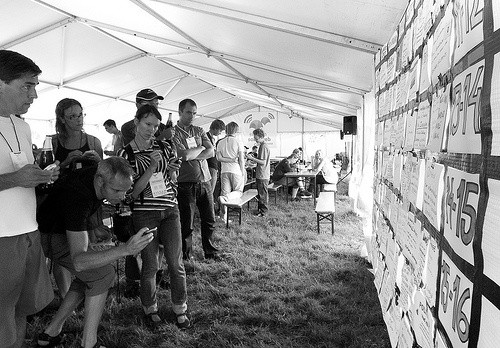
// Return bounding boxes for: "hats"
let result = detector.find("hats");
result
[136,88,164,103]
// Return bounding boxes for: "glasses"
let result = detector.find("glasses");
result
[61,113,86,120]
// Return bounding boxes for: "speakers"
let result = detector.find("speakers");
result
[344,115,357,135]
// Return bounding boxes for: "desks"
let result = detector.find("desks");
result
[284,164,321,205]
[246,161,279,181]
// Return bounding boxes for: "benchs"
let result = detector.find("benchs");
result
[221,171,337,236]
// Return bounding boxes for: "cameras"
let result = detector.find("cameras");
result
[144,227,158,239]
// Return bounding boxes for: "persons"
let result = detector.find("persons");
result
[0,50,60,348]
[36,88,187,348]
[206,119,258,223]
[174,99,226,263]
[246,128,270,218]
[272,147,338,202]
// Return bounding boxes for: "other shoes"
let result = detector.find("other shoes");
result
[147,313,162,324]
[205,252,224,261]
[79,342,107,348]
[291,198,302,202]
[38,332,65,345]
[126,283,140,297]
[176,312,191,328]
[300,191,312,196]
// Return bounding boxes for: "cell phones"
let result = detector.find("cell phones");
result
[43,163,57,171]
[81,150,94,158]
[171,157,183,164]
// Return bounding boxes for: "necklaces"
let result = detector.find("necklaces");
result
[62,131,83,154]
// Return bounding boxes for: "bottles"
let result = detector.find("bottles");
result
[166,113,174,142]
[297,162,299,172]
[40,135,54,189]
